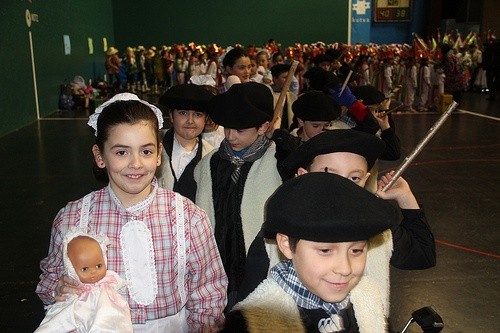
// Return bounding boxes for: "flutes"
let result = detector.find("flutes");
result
[375,101,459,192]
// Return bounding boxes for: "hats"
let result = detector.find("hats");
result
[292,129,384,170]
[207,82,274,129]
[159,83,215,117]
[291,91,341,122]
[350,85,385,105]
[262,171,401,243]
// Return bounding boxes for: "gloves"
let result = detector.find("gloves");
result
[333,86,355,106]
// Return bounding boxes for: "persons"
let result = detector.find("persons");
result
[217,171,400,333]
[33,225,135,333]
[35,92,229,333]
[288,89,352,142]
[59,27,500,115]
[269,64,295,129]
[228,129,436,308]
[199,86,226,149]
[158,83,216,191]
[327,82,358,129]
[172,82,306,288]
[350,84,400,160]
